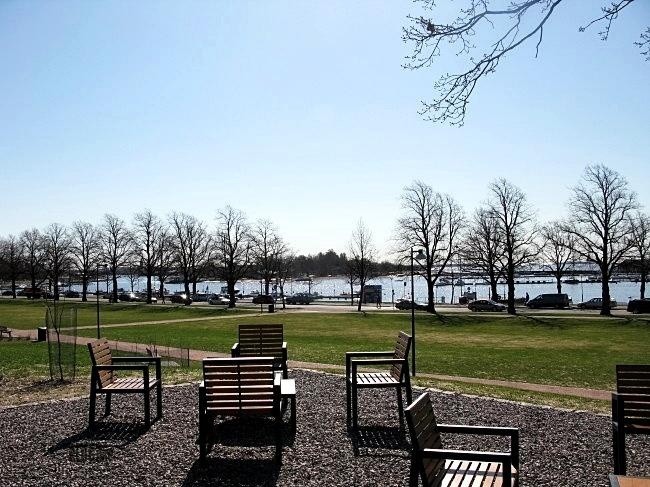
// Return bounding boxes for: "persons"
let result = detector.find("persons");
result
[526,292,528,302]
[343,291,347,302]
[162,295,166,305]
[376,294,381,308]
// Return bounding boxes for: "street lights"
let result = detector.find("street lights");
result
[411,247,425,377]
[96,263,109,339]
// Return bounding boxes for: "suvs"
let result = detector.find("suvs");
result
[284,291,314,305]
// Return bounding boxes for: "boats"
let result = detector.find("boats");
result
[338,290,360,297]
[394,268,466,287]
[532,269,650,285]
[16,275,284,300]
[294,274,314,281]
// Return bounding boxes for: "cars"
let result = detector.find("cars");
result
[1,290,13,297]
[251,295,276,305]
[576,297,617,310]
[135,295,158,304]
[395,299,429,311]
[169,293,194,305]
[208,296,230,305]
[466,298,506,312]
[626,296,650,314]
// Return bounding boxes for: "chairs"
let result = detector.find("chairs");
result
[228,321,291,378]
[82,334,162,432]
[606,359,650,472]
[401,389,526,487]
[194,356,287,461]
[343,328,417,445]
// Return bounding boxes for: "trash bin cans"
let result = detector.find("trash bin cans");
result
[269,305,274,313]
[38,327,47,341]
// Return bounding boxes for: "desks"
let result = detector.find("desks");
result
[607,471,647,487]
[271,368,297,422]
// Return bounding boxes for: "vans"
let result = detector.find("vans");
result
[526,292,571,309]
